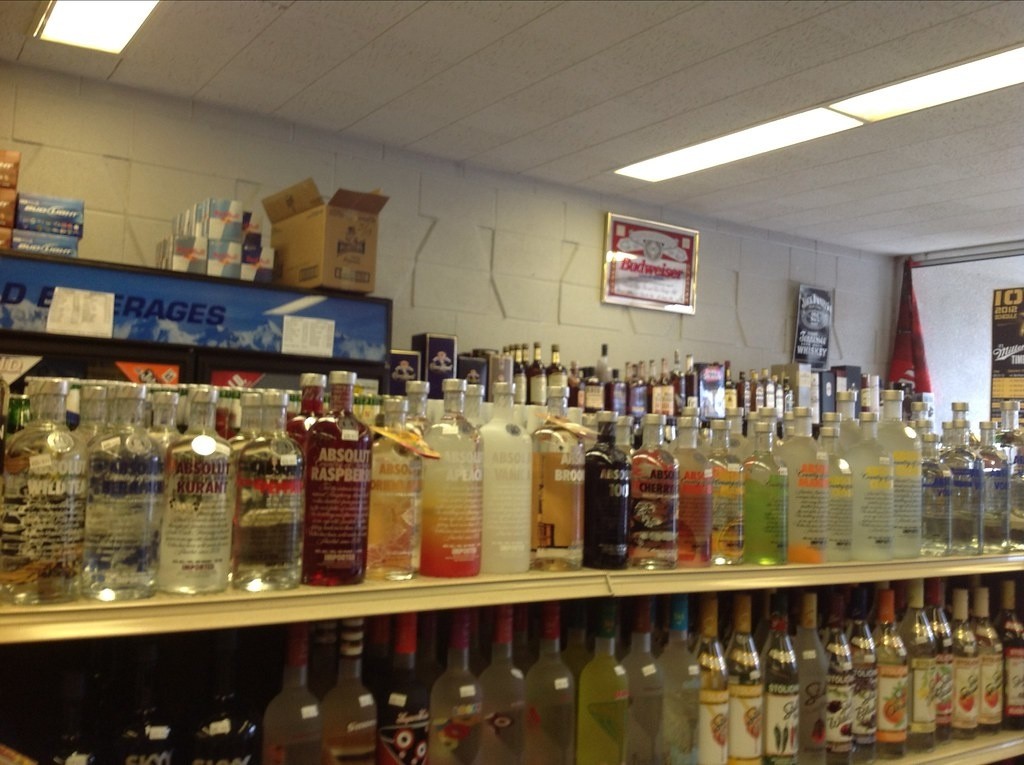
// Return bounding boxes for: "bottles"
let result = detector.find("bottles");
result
[0,563,1024,765]
[0,341,1024,602]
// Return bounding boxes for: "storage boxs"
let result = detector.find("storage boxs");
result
[0,227,12,252]
[457,356,488,402]
[390,348,421,396]
[15,190,85,238]
[11,229,79,258]
[0,188,18,228]
[412,333,458,400]
[0,149,22,189]
[161,196,257,283]
[261,177,391,293]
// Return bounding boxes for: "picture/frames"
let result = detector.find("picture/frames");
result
[598,212,700,316]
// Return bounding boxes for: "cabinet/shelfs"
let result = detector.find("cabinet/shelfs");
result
[0,546,1024,765]
